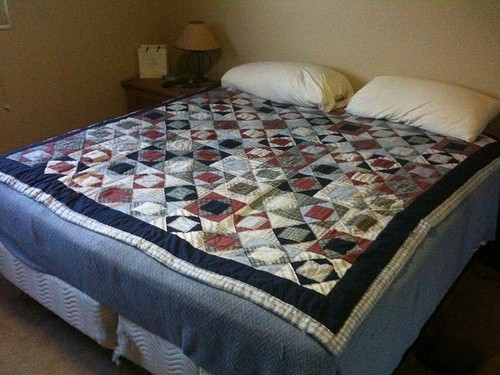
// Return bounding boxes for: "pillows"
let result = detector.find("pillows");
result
[220,60,356,114]
[344,73,498,145]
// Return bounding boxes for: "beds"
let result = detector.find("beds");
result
[0,88,500,375]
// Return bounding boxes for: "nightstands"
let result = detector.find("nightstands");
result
[119,75,221,113]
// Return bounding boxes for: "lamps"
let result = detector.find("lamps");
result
[174,20,220,89]
